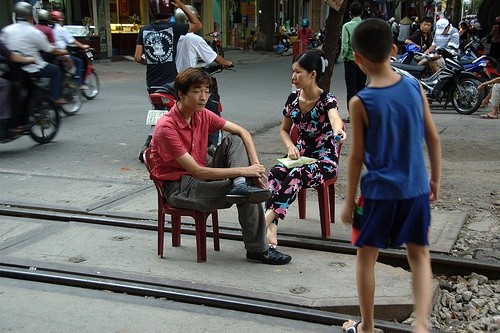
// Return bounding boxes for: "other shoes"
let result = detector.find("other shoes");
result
[49,97,70,106]
[343,116,350,123]
[0,122,25,134]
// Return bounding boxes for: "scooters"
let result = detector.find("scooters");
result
[276,25,326,52]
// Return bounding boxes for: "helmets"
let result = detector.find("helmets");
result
[50,11,65,21]
[13,1,33,20]
[38,10,50,21]
[302,19,309,26]
[175,5,200,24]
[148,0,175,18]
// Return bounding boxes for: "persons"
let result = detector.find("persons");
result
[133,0,232,163]
[264,48,346,249]
[341,19,441,333]
[298,19,315,50]
[386,16,500,119]
[149,67,292,265]
[0,1,90,132]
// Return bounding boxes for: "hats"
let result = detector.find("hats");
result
[436,18,449,34]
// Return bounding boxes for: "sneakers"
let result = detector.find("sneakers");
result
[246,245,292,265]
[226,183,271,204]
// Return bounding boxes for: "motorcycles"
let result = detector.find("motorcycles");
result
[389,37,498,115]
[0,43,99,144]
[146,63,237,148]
[203,31,225,58]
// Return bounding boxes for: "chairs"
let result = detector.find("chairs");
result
[291,119,346,238]
[142,147,221,262]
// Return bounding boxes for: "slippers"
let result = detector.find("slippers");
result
[343,320,361,333]
[480,113,499,119]
